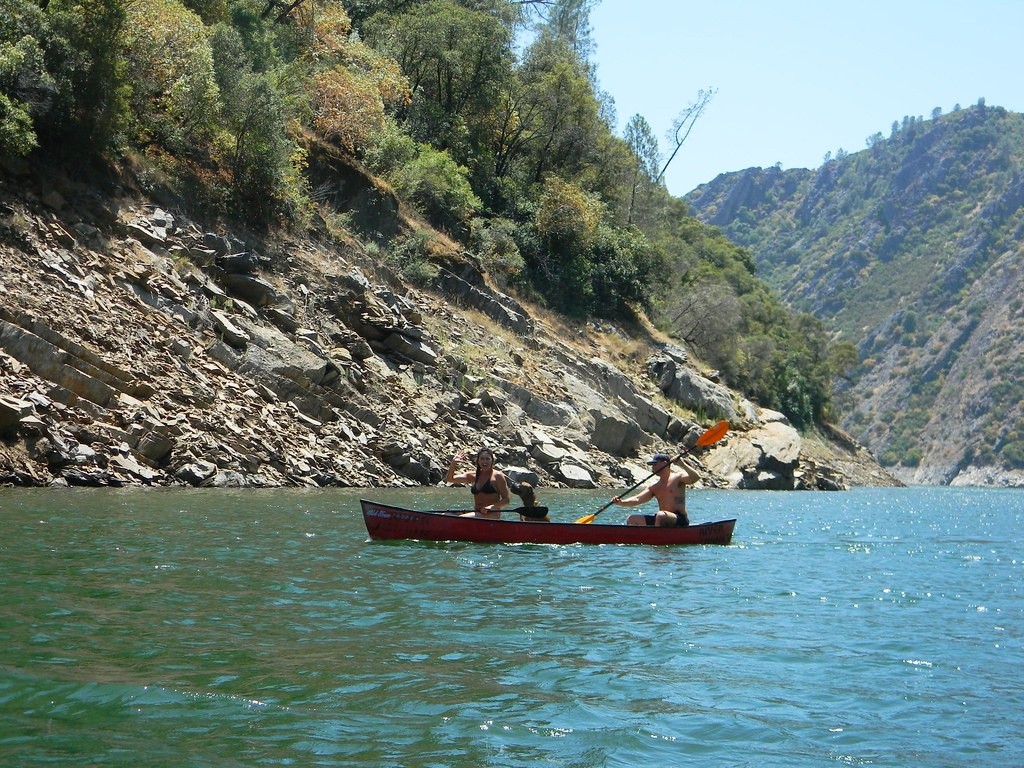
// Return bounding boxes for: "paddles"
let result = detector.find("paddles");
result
[428,506,549,518]
[574,420,730,524]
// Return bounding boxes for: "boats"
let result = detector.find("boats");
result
[359,499,737,545]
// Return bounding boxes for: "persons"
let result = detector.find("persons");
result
[612,454,701,527]
[443,447,510,518]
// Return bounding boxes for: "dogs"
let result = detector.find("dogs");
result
[510,481,551,522]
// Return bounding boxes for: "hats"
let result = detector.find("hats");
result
[647,454,669,465]
